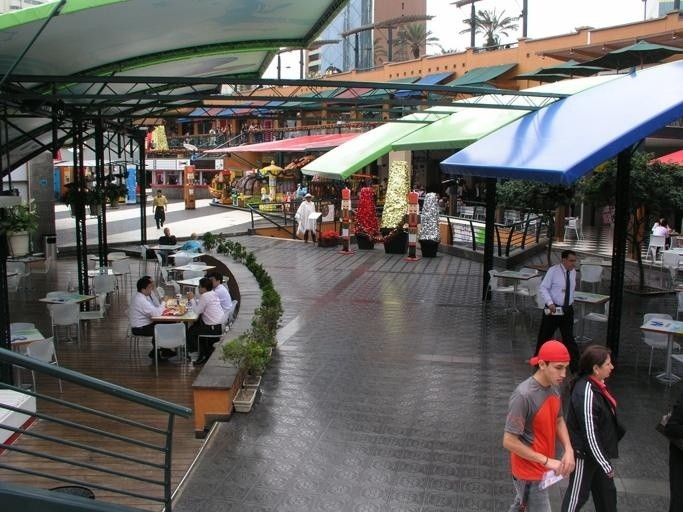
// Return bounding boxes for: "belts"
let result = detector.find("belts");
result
[205,324,221,330]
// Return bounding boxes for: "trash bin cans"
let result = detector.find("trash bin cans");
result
[44,234,57,260]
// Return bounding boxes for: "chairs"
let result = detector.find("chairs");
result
[488,267,543,308]
[635,229,682,386]
[458,198,583,242]
[582,300,610,344]
[554,314,579,341]
[579,265,605,293]
[6,246,238,394]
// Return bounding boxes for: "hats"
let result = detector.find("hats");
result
[529,340,572,367]
[304,193,314,198]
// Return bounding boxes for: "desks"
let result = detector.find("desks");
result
[573,291,610,346]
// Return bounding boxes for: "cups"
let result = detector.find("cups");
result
[176,294,182,304]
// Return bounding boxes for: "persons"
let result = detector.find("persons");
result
[446,179,484,202]
[294,193,318,244]
[159,226,178,245]
[153,190,169,228]
[561,344,620,512]
[184,233,201,250]
[208,124,231,146]
[502,339,576,512]
[651,217,673,253]
[128,271,232,365]
[239,121,265,144]
[534,250,583,374]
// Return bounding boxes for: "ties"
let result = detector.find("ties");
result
[563,270,570,316]
[149,295,153,302]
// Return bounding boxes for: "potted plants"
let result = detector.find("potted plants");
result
[204,231,283,413]
[459,9,523,51]
[4,204,40,258]
[392,24,445,58]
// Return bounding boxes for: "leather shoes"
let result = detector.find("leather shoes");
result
[149,349,168,361]
[169,350,177,358]
[195,352,209,365]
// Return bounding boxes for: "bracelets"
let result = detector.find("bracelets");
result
[542,455,549,467]
